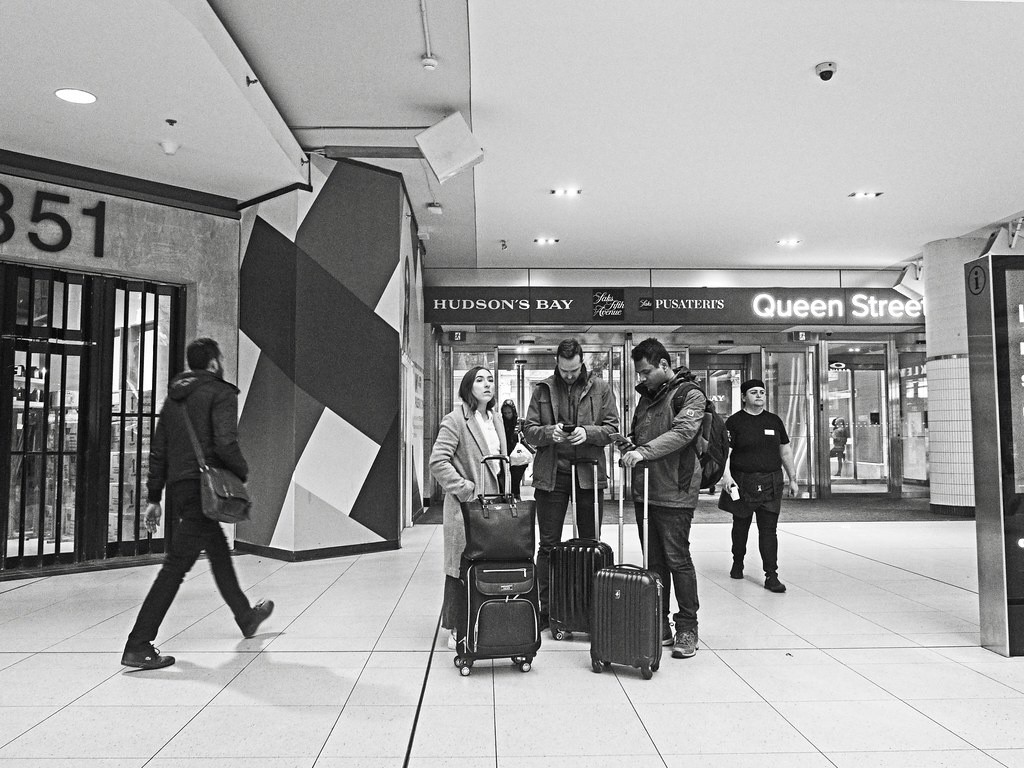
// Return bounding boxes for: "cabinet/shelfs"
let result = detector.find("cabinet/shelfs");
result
[25,406,151,543]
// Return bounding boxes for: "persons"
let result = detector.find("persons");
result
[120,338,274,670]
[829,417,847,477]
[501,399,537,502]
[721,379,798,593]
[523,339,619,631]
[614,339,707,659]
[428,367,513,650]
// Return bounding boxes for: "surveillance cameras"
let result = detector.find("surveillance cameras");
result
[826,331,832,336]
[815,62,837,82]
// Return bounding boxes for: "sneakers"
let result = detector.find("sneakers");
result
[672,625,700,658]
[121,643,174,670]
[662,628,673,645]
[730,560,744,579]
[242,599,274,639]
[764,576,786,593]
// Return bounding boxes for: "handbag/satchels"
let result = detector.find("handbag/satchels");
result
[509,433,534,466]
[461,493,536,561]
[200,467,251,525]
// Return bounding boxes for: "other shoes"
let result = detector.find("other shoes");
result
[710,487,716,494]
[842,453,846,460]
[834,473,841,476]
[448,628,457,652]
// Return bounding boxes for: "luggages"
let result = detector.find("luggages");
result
[549,458,615,642]
[454,455,542,678]
[589,459,664,680]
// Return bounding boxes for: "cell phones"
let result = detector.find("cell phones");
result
[562,425,575,434]
[608,433,633,445]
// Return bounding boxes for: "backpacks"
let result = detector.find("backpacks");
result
[674,382,729,489]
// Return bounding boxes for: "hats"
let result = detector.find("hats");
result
[740,379,763,392]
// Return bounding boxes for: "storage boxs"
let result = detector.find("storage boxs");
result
[44,390,153,538]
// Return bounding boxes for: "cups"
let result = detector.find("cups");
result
[730,487,740,501]
